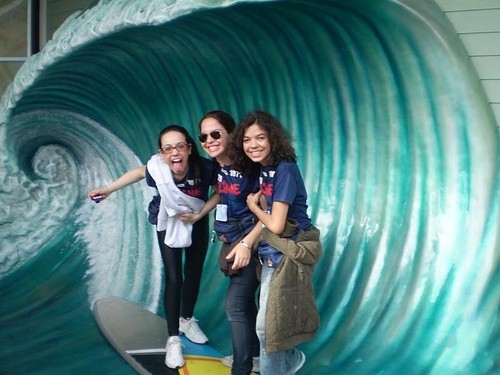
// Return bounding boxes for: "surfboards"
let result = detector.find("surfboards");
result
[93,296,258,375]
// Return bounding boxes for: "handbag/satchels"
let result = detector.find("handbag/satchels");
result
[213,214,257,244]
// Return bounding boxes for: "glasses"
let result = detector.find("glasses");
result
[162,142,190,154]
[198,129,225,145]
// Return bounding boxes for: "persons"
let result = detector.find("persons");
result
[226,109,322,375]
[199,110,262,375]
[86,124,221,369]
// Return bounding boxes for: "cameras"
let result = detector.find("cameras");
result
[90,194,107,203]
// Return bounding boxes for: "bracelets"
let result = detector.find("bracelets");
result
[240,241,252,250]
[104,186,111,195]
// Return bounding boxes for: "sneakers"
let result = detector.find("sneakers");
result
[165,337,185,368]
[177,315,208,343]
[220,351,261,371]
